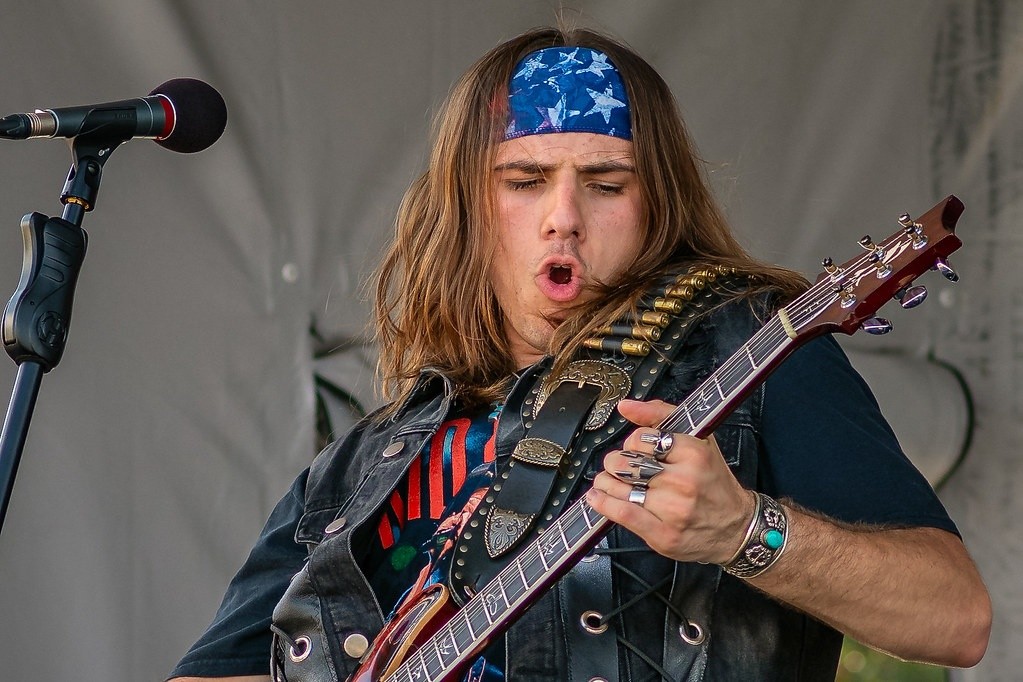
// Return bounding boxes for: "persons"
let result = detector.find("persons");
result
[163,25,993,682]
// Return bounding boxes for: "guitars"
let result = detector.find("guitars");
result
[344,194,966,682]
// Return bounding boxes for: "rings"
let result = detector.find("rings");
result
[614,428,675,507]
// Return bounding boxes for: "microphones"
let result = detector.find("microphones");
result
[0,77,228,155]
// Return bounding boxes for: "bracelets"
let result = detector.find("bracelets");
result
[719,490,789,580]
